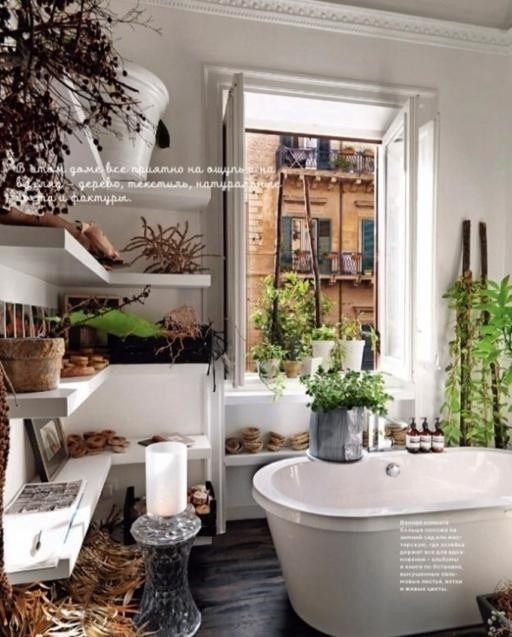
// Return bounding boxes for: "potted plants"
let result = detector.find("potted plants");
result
[250,273,395,464]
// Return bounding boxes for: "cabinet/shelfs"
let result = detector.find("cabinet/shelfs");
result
[0,48,211,586]
[224,396,420,467]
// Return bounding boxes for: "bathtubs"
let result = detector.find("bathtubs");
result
[251,448,512,637]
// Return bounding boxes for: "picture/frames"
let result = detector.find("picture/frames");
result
[24,419,70,485]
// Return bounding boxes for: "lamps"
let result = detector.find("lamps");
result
[129,443,203,637]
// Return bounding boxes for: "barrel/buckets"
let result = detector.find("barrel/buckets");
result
[309,405,365,462]
[72,62,169,182]
